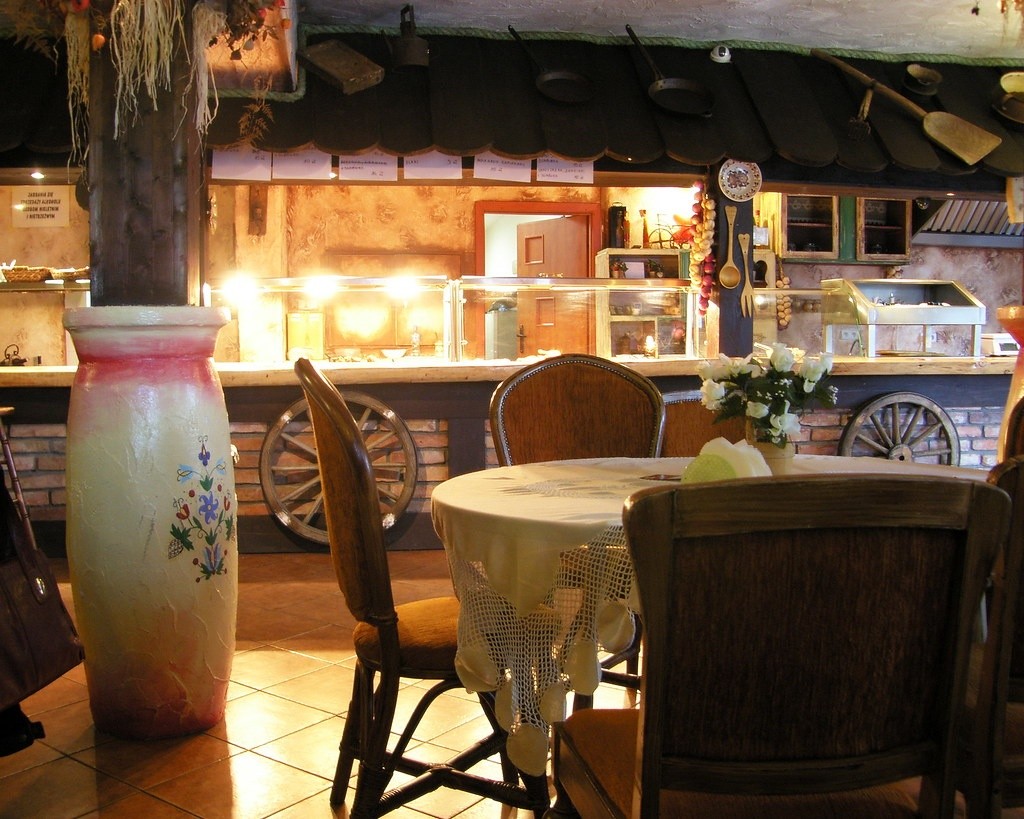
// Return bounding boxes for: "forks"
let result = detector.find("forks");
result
[736,233,758,318]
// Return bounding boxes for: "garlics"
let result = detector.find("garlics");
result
[775,276,791,327]
[792,302,820,311]
[688,198,716,291]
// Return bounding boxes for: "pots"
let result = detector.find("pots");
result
[622,22,720,120]
[504,25,597,105]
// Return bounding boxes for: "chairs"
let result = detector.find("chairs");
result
[296,358,552,818]
[550,398,1024,819]
[489,350,664,467]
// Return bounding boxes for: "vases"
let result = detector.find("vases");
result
[744,413,796,476]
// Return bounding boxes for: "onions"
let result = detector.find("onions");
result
[689,179,703,245]
[698,254,714,315]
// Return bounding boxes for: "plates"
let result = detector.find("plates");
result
[717,158,762,203]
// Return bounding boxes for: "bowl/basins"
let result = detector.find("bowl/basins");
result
[993,72,1024,123]
[380,349,408,358]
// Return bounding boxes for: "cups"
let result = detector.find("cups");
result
[904,63,942,94]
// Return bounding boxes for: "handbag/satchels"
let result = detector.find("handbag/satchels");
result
[0,479,84,710]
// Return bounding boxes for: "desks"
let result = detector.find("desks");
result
[432,457,991,768]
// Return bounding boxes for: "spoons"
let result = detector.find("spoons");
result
[719,205,741,289]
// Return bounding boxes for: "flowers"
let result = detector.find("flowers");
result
[697,341,838,448]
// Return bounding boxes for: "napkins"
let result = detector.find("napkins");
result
[700,437,774,477]
